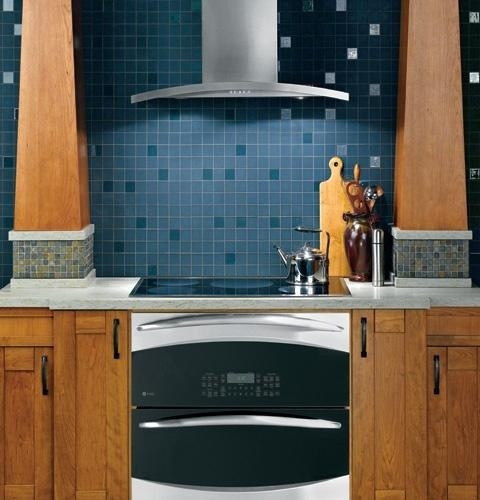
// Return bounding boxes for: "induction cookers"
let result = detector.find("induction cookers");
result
[131,273,352,298]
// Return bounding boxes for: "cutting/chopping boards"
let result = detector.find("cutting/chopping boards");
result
[318,154,354,279]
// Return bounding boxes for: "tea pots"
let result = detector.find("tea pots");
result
[274,226,331,287]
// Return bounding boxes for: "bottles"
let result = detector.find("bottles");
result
[371,228,384,286]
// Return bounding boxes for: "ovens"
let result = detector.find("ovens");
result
[130,312,353,500]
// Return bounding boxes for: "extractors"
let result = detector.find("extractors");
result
[129,1,350,107]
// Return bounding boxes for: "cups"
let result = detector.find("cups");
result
[341,211,374,283]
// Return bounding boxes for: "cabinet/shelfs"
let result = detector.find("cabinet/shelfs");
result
[350,304,427,500]
[1,306,52,500]
[425,304,480,500]
[51,310,130,500]
